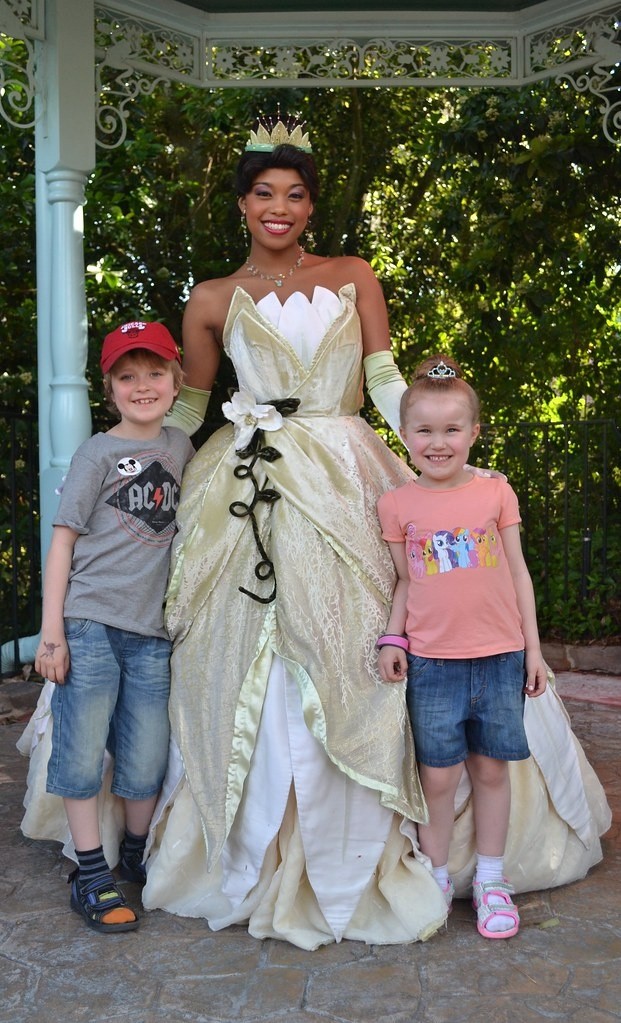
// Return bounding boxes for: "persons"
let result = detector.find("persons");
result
[35,322,197,934]
[378,356,547,938]
[16,120,611,952]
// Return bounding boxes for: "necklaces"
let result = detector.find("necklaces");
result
[246,246,304,287]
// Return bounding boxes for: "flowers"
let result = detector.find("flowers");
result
[217,389,285,450]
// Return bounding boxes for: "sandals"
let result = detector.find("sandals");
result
[430,874,455,915]
[470,872,521,939]
[118,840,151,884]
[66,866,141,932]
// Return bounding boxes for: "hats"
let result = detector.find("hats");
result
[100,321,181,374]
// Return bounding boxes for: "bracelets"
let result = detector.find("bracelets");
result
[377,634,409,651]
